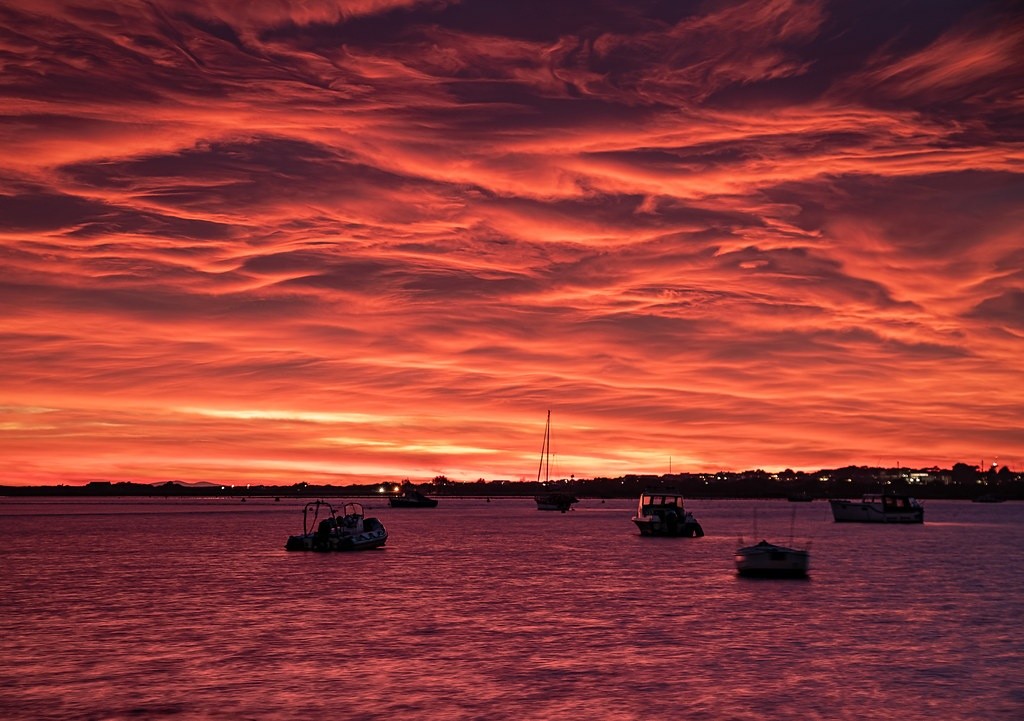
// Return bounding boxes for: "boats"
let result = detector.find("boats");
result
[788,491,812,502]
[827,494,927,524]
[632,493,704,537]
[732,540,810,576]
[285,499,388,552]
[388,491,438,507]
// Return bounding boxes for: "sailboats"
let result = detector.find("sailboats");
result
[534,410,581,510]
